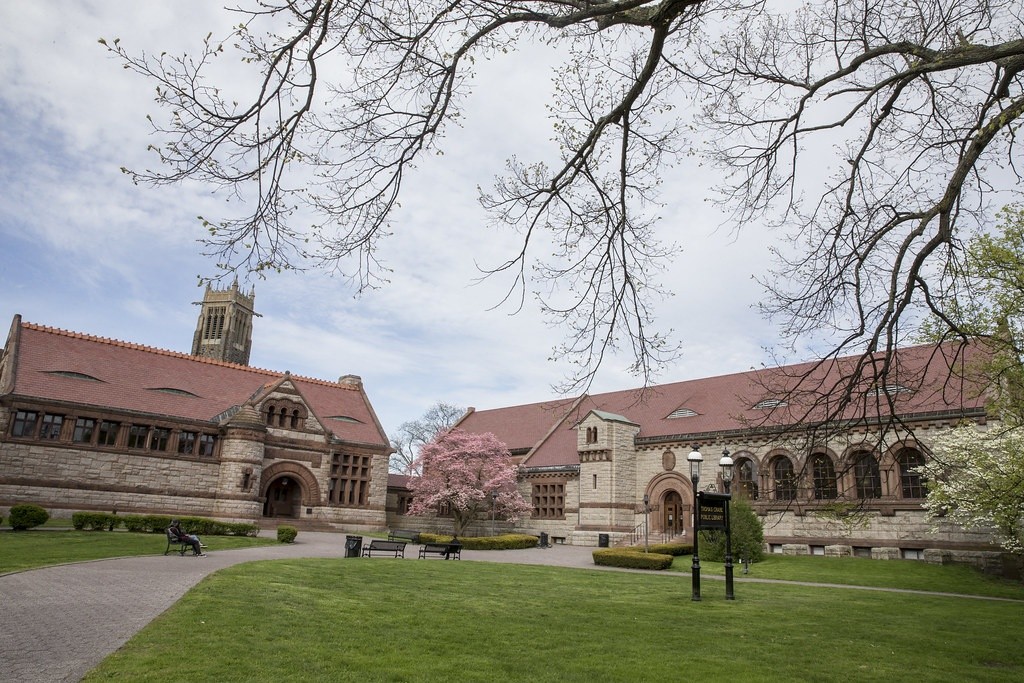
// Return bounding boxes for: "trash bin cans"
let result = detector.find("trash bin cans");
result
[345,535,363,558]
[598,532,609,548]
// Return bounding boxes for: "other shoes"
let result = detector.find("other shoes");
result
[445,558,450,560]
[200,544,207,548]
[440,553,445,555]
[196,554,206,557]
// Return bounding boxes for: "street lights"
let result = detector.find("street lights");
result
[718,445,735,600]
[687,438,704,601]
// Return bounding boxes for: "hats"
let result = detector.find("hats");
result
[173,520,178,523]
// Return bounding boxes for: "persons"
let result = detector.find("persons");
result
[168,518,207,556]
[440,533,461,560]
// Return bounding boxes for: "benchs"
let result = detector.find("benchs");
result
[164,529,193,556]
[418,543,463,561]
[552,537,565,544]
[360,539,407,558]
[387,529,421,545]
